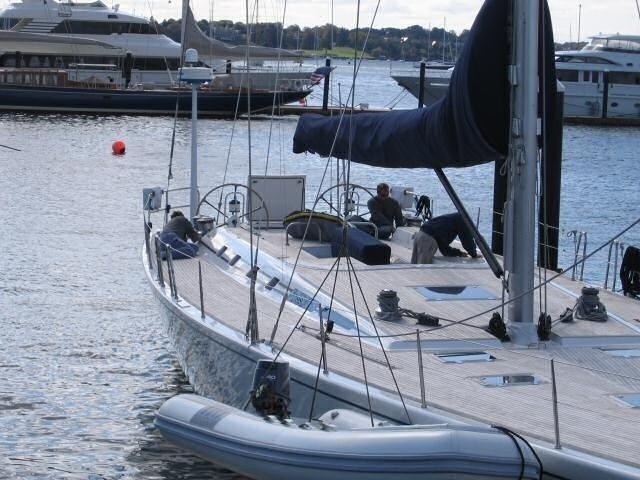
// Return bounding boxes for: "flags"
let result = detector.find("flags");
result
[310,66,334,86]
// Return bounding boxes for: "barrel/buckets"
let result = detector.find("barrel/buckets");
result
[111,141,125,154]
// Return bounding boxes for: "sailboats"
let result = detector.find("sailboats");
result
[414,15,459,70]
[136,0,640,480]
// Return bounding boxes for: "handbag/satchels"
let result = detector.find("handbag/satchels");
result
[284,212,343,241]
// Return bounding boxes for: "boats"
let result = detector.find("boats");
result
[0,0,314,92]
[0,67,316,124]
[386,30,640,125]
[147,359,545,480]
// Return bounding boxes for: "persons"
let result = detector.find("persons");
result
[410,211,483,265]
[122,51,133,89]
[156,210,202,260]
[366,182,403,241]
